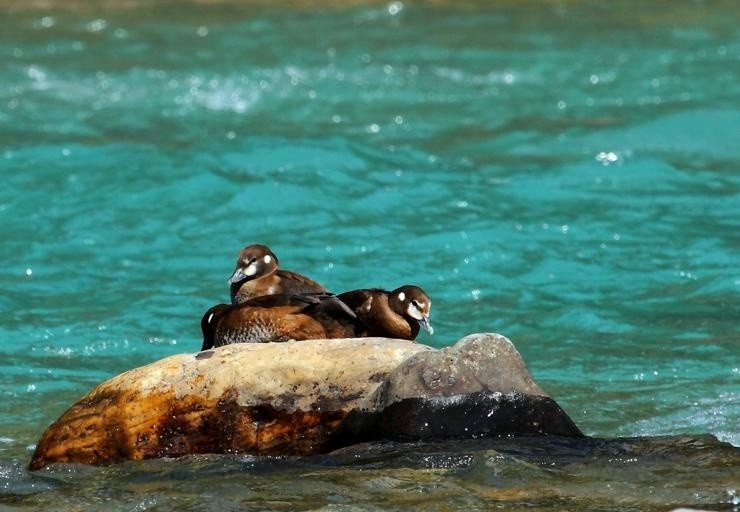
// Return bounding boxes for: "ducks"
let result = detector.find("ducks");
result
[227,244,331,304]
[200,293,357,350]
[305,283,434,340]
